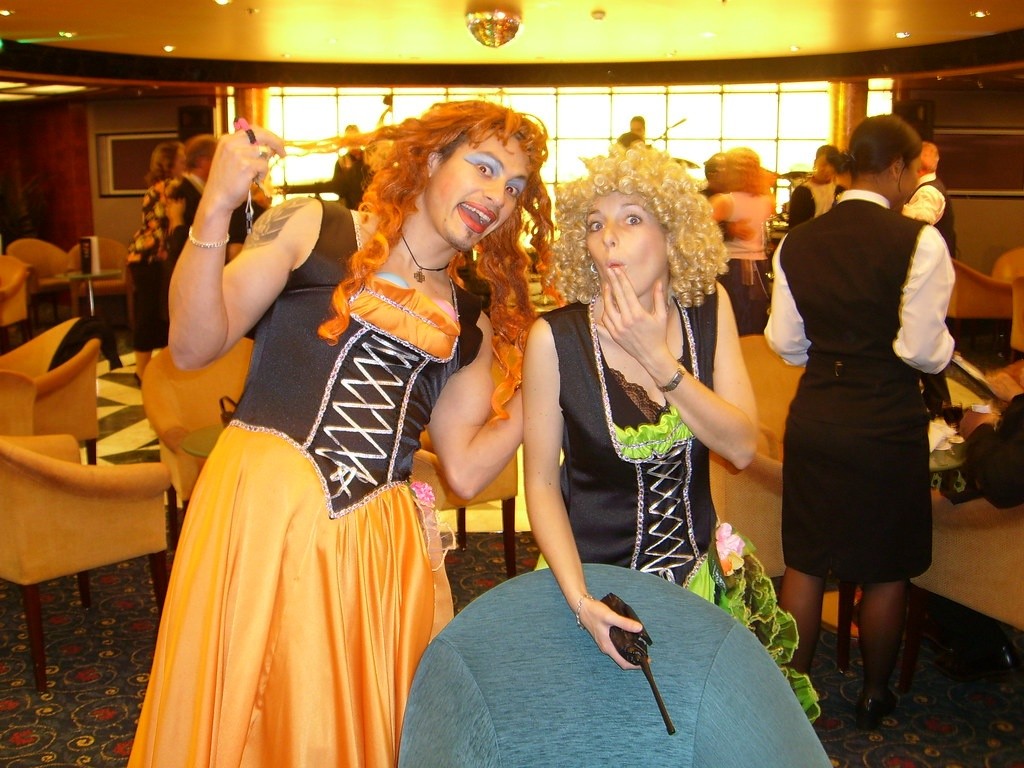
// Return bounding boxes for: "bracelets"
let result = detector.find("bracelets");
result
[575,593,595,631]
[657,365,686,393]
[188,225,230,249]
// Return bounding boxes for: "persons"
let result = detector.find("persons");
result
[521,141,761,672]
[762,114,958,732]
[120,117,956,351]
[958,358,1024,508]
[123,101,569,768]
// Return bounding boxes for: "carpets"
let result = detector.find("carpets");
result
[0,530,1024,767]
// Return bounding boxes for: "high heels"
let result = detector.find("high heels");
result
[855,689,897,727]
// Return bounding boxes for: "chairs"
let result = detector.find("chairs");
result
[410,434,517,577]
[399,563,831,768]
[0,239,253,690]
[949,246,1023,366]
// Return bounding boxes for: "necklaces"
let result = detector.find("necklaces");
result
[399,226,449,283]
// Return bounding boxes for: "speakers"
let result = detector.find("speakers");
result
[178,106,212,141]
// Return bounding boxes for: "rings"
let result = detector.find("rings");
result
[259,145,268,159]
